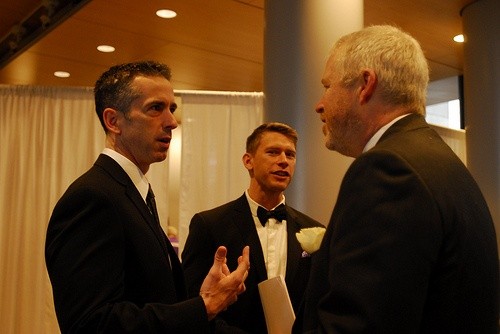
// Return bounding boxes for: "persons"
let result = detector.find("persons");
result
[292,23,500,334]
[179,121,325,334]
[45,62,250,334]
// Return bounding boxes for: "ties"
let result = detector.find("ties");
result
[146,182,160,233]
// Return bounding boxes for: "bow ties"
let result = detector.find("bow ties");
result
[257,203,288,227]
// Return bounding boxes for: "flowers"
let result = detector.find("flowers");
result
[296,226,327,258]
